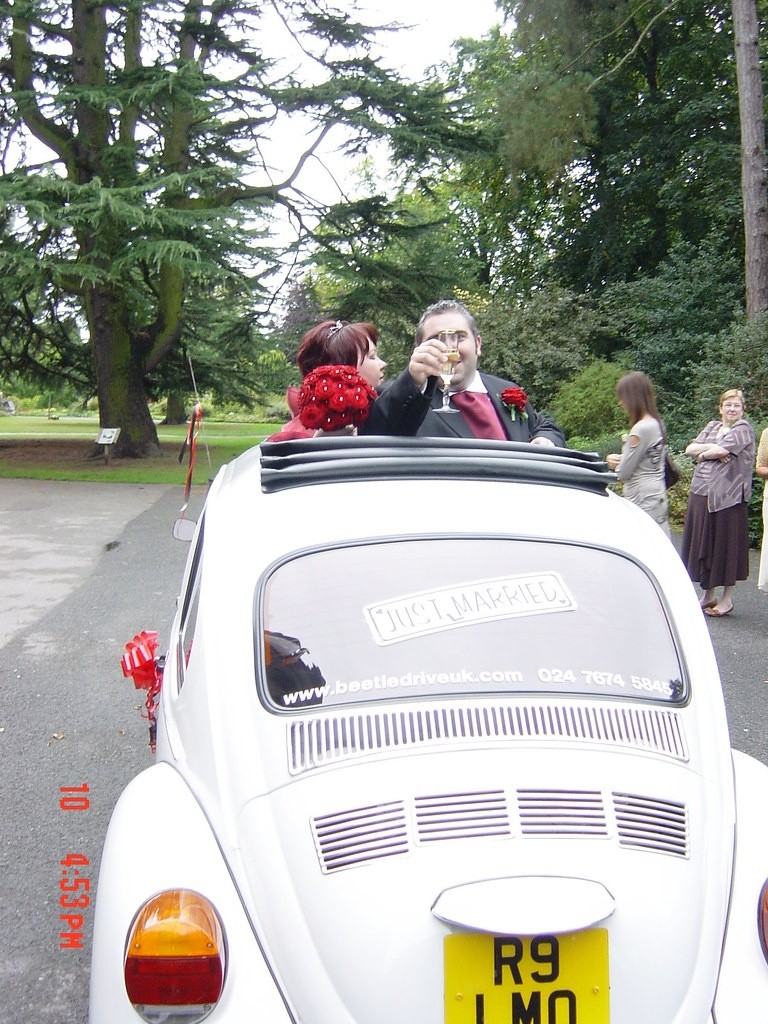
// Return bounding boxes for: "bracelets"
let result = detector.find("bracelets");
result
[701,452,705,462]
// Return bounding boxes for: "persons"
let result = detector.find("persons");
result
[357,300,566,450]
[606,371,671,543]
[755,426,768,592]
[679,389,755,617]
[260,319,388,444]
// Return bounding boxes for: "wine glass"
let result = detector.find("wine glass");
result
[431,329,461,415]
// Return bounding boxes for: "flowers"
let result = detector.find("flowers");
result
[500,386,530,423]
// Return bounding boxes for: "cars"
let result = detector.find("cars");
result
[87,424,768,1024]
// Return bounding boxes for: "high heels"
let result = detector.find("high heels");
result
[700,598,734,617]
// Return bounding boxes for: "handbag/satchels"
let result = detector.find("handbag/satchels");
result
[658,419,681,490]
